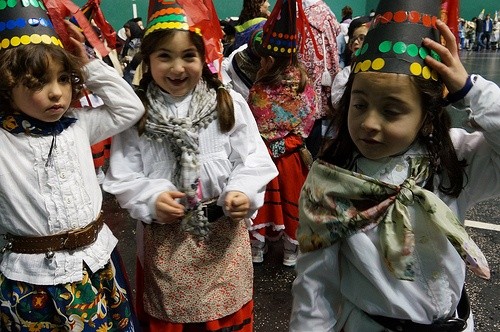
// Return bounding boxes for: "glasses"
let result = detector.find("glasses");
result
[351,35,366,44]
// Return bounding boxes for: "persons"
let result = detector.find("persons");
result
[103,1,279,331]
[0,0,145,332]
[289,0,500,332]
[68,1,500,266]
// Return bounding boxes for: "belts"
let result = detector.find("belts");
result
[363,284,470,332]
[202,200,224,223]
[0,207,105,259]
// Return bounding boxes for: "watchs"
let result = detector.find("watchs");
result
[446,74,478,103]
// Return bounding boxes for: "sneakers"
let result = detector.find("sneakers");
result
[252,247,264,263]
[283,249,298,266]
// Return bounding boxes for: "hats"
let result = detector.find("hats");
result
[351,0,444,85]
[142,0,203,41]
[68,0,101,33]
[0,0,65,49]
[255,0,296,57]
